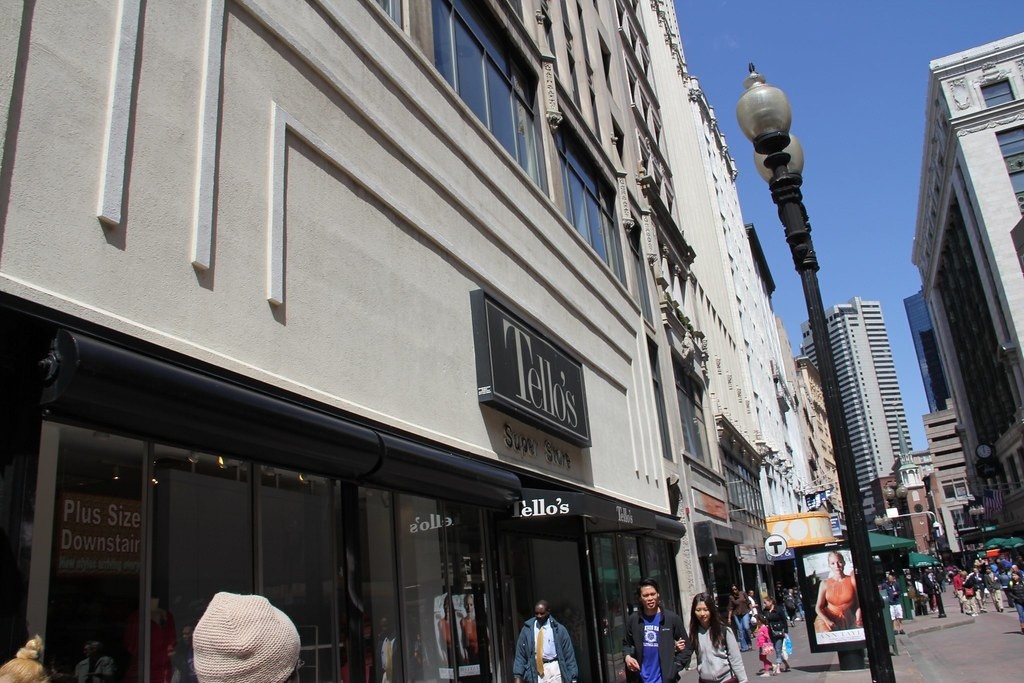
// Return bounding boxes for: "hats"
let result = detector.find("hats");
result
[192,591,302,683]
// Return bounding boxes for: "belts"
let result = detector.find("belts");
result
[543,658,558,663]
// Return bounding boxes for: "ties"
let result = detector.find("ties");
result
[536,627,544,676]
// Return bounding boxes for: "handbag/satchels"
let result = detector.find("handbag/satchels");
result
[761,643,774,655]
[965,588,974,596]
[781,633,793,661]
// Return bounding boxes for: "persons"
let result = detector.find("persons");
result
[0,634,52,683]
[165,624,194,683]
[58,641,113,683]
[953,556,1024,634]
[728,582,806,677]
[460,595,478,664]
[814,551,861,633]
[623,577,692,683]
[374,616,430,683]
[193,592,301,683]
[675,592,748,683]
[882,567,946,636]
[125,597,177,683]
[436,596,450,655]
[513,599,578,683]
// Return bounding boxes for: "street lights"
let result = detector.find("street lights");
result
[735,61,899,683]
[968,506,988,542]
[883,482,910,536]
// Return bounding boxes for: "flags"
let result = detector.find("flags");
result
[983,489,1003,520]
[805,487,833,511]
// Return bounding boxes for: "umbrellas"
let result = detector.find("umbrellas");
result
[909,551,940,566]
[977,537,1024,557]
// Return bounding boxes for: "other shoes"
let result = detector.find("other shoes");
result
[760,673,770,678]
[783,664,789,671]
[772,665,777,675]
[776,663,780,674]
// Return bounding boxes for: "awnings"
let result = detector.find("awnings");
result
[513,488,655,533]
